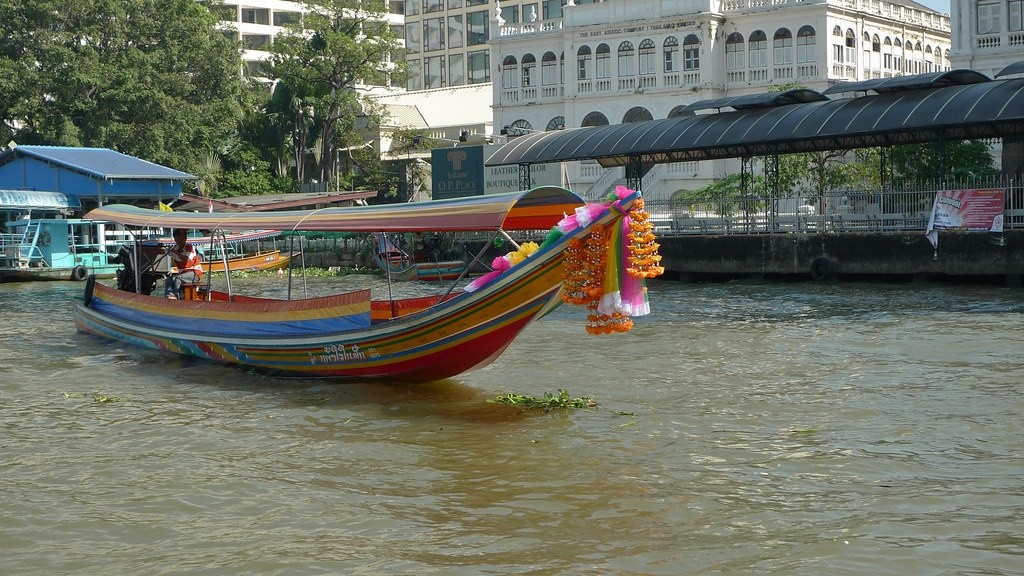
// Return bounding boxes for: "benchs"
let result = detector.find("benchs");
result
[180,282,208,301]
[6,244,29,268]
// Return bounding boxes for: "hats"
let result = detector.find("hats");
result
[173,232,187,242]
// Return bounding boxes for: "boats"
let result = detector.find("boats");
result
[0,215,135,280]
[370,232,465,281]
[76,186,643,384]
[140,229,301,273]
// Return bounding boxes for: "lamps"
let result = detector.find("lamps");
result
[459,132,470,142]
[413,135,425,144]
[502,125,513,135]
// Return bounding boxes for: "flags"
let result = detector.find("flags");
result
[208,199,216,213]
[160,202,173,212]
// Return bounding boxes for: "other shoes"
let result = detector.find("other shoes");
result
[166,294,177,300]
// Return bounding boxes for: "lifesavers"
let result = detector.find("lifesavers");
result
[73,265,89,281]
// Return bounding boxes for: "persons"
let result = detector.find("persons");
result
[164,229,203,300]
